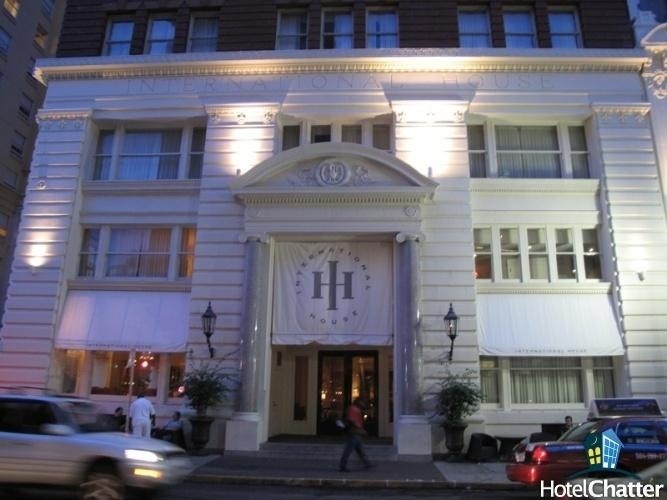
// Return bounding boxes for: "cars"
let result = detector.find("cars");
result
[506,415,667,485]
[0,394,193,500]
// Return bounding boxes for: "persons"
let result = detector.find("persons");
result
[337,397,377,473]
[156,411,183,442]
[559,416,574,437]
[107,406,126,430]
[129,392,155,438]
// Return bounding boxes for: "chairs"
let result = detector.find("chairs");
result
[167,428,188,451]
[467,433,498,463]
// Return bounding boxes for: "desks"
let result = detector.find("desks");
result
[495,435,527,462]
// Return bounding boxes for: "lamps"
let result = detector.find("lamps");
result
[202,300,216,358]
[444,302,459,359]
[170,348,244,455]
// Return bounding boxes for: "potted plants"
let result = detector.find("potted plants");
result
[421,363,488,462]
